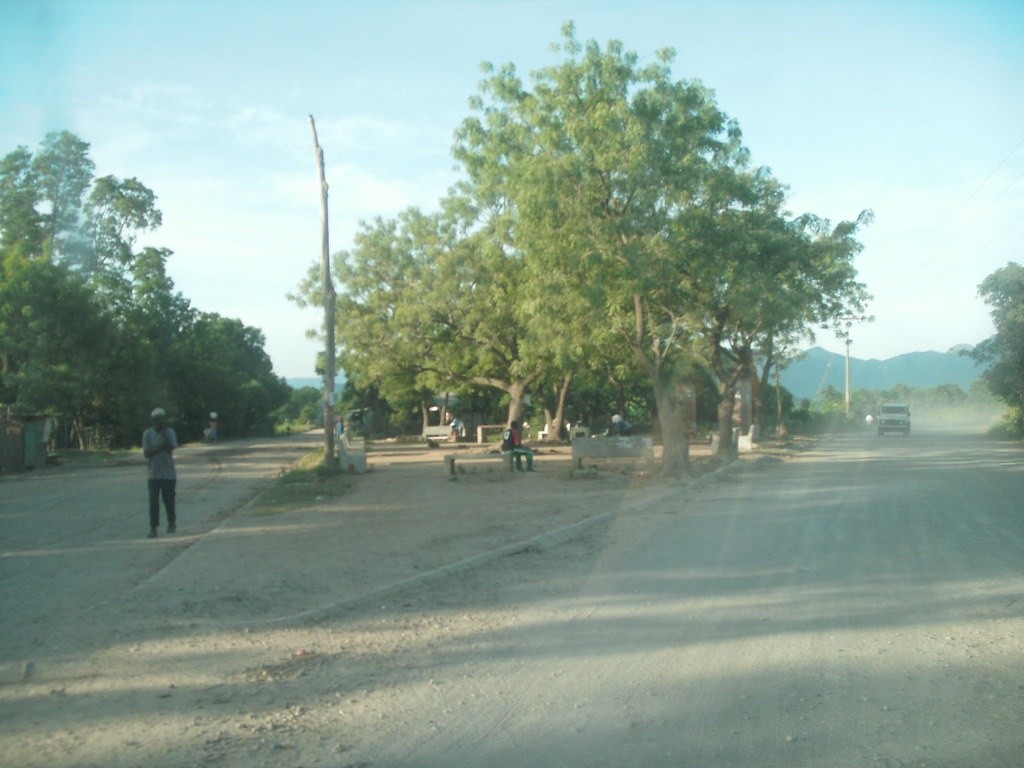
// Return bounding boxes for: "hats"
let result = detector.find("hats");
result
[150,408,166,416]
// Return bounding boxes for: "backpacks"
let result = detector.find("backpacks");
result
[503,430,515,450]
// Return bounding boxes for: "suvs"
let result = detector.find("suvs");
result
[876,403,911,436]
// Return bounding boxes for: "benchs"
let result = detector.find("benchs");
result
[572,437,654,469]
[337,430,366,473]
[444,453,513,476]
[421,424,453,441]
[537,431,549,441]
[737,425,760,449]
[711,427,739,453]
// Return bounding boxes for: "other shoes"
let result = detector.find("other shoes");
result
[148,530,157,538]
[167,522,177,534]
[527,467,535,472]
[516,468,524,471]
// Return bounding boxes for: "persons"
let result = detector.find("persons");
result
[336,416,342,439]
[605,414,631,437]
[209,413,218,442]
[445,411,461,442]
[142,409,178,536]
[508,420,535,472]
